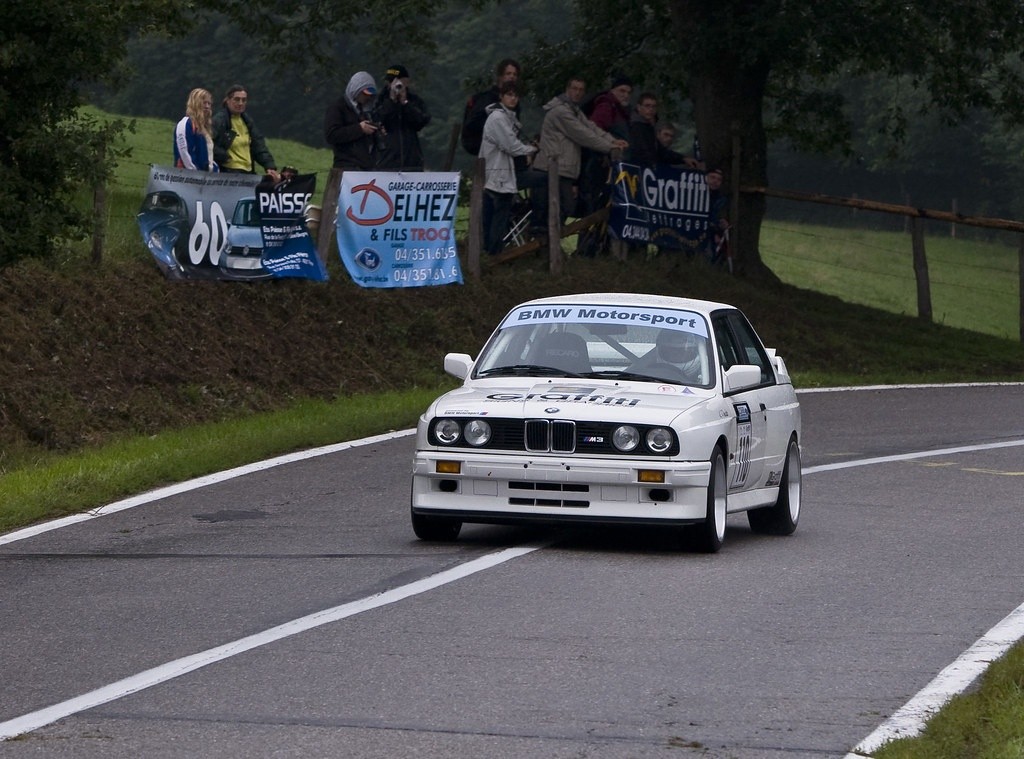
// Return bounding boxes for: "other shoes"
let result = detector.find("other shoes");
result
[498,260,513,266]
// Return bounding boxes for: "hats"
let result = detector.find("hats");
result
[381,64,410,82]
[610,67,634,91]
[362,87,376,96]
[706,166,726,180]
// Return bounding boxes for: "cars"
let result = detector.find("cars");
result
[409,291,804,556]
[137,188,189,260]
[219,196,273,279]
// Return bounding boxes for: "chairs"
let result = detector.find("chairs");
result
[528,332,595,374]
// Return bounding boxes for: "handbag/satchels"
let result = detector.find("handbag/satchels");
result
[510,140,528,173]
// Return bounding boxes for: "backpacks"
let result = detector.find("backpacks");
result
[463,91,499,156]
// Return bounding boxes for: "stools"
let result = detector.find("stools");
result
[498,203,534,248]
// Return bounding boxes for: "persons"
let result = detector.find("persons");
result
[476,80,542,255]
[373,63,432,172]
[626,91,656,157]
[532,73,630,244]
[632,327,710,383]
[209,85,280,180]
[461,57,527,192]
[700,167,734,274]
[635,122,703,169]
[573,70,635,258]
[323,67,387,171]
[173,86,223,175]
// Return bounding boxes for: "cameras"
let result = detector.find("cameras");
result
[370,121,388,151]
[394,82,402,94]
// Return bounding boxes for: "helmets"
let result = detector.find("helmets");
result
[655,328,701,378]
